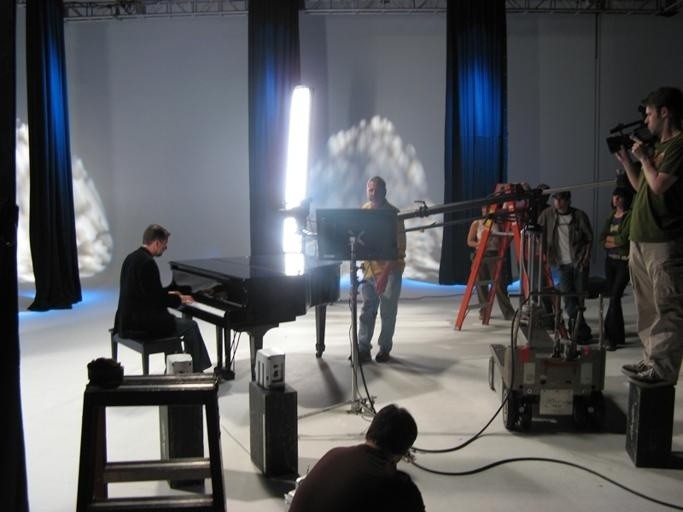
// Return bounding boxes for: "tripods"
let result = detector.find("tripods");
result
[299,263,416,463]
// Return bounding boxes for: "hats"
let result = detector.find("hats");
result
[552,191,571,198]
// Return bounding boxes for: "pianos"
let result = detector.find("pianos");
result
[168,254,342,382]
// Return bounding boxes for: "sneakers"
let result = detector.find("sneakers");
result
[627,368,677,388]
[620,360,652,376]
[348,351,371,362]
[376,351,389,362]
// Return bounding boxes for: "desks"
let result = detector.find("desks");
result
[77,374,225,512]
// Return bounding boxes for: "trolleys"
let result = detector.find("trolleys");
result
[487,227,608,431]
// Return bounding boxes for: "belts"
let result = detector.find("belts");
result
[609,253,627,261]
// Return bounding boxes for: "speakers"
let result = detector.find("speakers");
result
[249,381,298,478]
[626,383,675,467]
[167,353,193,376]
[255,348,285,388]
[159,404,204,488]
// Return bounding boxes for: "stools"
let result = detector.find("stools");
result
[109,329,187,376]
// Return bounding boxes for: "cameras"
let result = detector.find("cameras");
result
[605,120,652,153]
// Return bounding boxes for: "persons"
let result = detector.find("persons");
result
[347,176,406,362]
[288,403,426,511]
[596,185,635,351]
[467,205,519,320]
[109,223,212,375]
[613,85,683,387]
[535,190,594,344]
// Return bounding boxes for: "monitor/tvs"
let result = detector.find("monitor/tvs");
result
[317,209,397,260]
